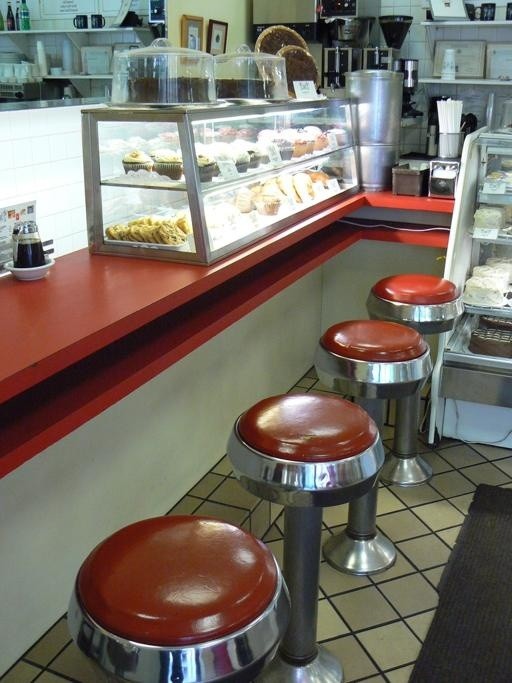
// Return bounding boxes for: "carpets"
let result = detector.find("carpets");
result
[408,482,512,682]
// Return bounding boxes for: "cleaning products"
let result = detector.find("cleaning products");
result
[18,0,29,30]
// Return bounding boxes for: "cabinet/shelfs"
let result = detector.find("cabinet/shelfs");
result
[418,18,511,85]
[428,126,512,452]
[81,96,362,266]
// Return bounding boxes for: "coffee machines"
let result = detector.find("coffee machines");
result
[362,14,424,118]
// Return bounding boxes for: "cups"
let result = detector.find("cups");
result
[441,48,457,81]
[36,40,48,76]
[73,15,88,29]
[0,62,39,83]
[63,40,73,74]
[91,15,105,28]
[473,3,496,21]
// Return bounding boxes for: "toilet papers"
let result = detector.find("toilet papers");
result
[61,39,73,72]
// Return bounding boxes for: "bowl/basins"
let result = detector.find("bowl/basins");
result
[50,67,62,75]
[4,256,56,282]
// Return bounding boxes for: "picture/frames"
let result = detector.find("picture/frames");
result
[179,13,228,65]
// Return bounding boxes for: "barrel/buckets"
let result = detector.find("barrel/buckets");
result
[343,68,403,191]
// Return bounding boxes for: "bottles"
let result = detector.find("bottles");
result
[7,0,30,31]
[12,220,46,268]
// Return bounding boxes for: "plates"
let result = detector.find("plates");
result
[0,52,25,63]
